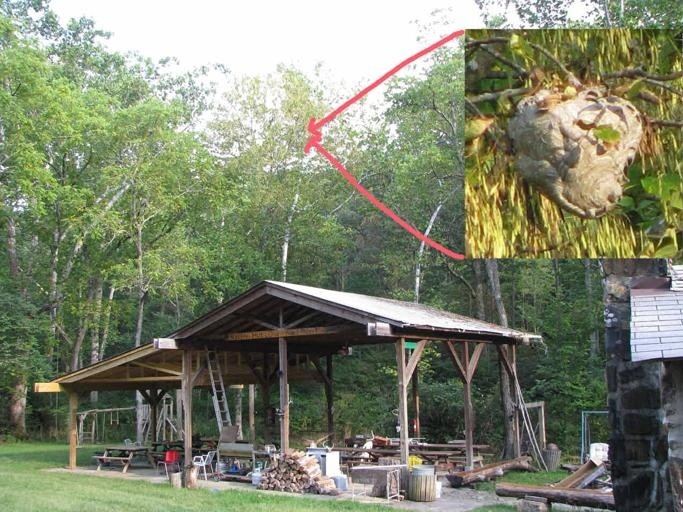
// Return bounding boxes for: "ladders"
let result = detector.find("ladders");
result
[205,345,233,435]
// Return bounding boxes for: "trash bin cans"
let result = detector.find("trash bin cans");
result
[410,464,436,502]
[542,443,562,472]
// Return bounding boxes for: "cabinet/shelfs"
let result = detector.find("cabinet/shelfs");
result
[216,442,276,481]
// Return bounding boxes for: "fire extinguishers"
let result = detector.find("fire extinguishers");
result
[413,419,417,432]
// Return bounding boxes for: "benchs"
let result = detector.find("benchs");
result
[136,451,166,456]
[92,455,129,461]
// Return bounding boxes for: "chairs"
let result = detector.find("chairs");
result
[191,450,216,481]
[156,450,181,479]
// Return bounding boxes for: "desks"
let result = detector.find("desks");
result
[330,442,490,480]
[96,447,157,473]
[152,441,198,447]
[350,467,400,503]
